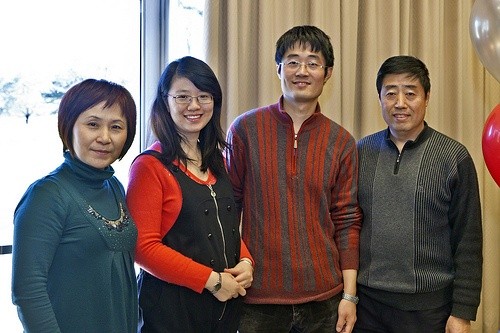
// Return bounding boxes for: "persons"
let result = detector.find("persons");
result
[225,25,363,333]
[356,55,484,333]
[126,56,255,333]
[11,79,139,333]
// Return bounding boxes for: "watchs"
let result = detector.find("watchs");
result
[212,272,222,295]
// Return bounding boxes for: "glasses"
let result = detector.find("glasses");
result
[166,93,213,104]
[278,60,327,72]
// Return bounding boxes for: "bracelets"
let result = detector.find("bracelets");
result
[240,260,254,272]
[342,293,359,304]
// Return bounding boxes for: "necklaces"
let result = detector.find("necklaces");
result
[187,153,199,167]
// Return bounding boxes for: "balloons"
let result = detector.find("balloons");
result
[482,103,500,188]
[469,0,500,83]
